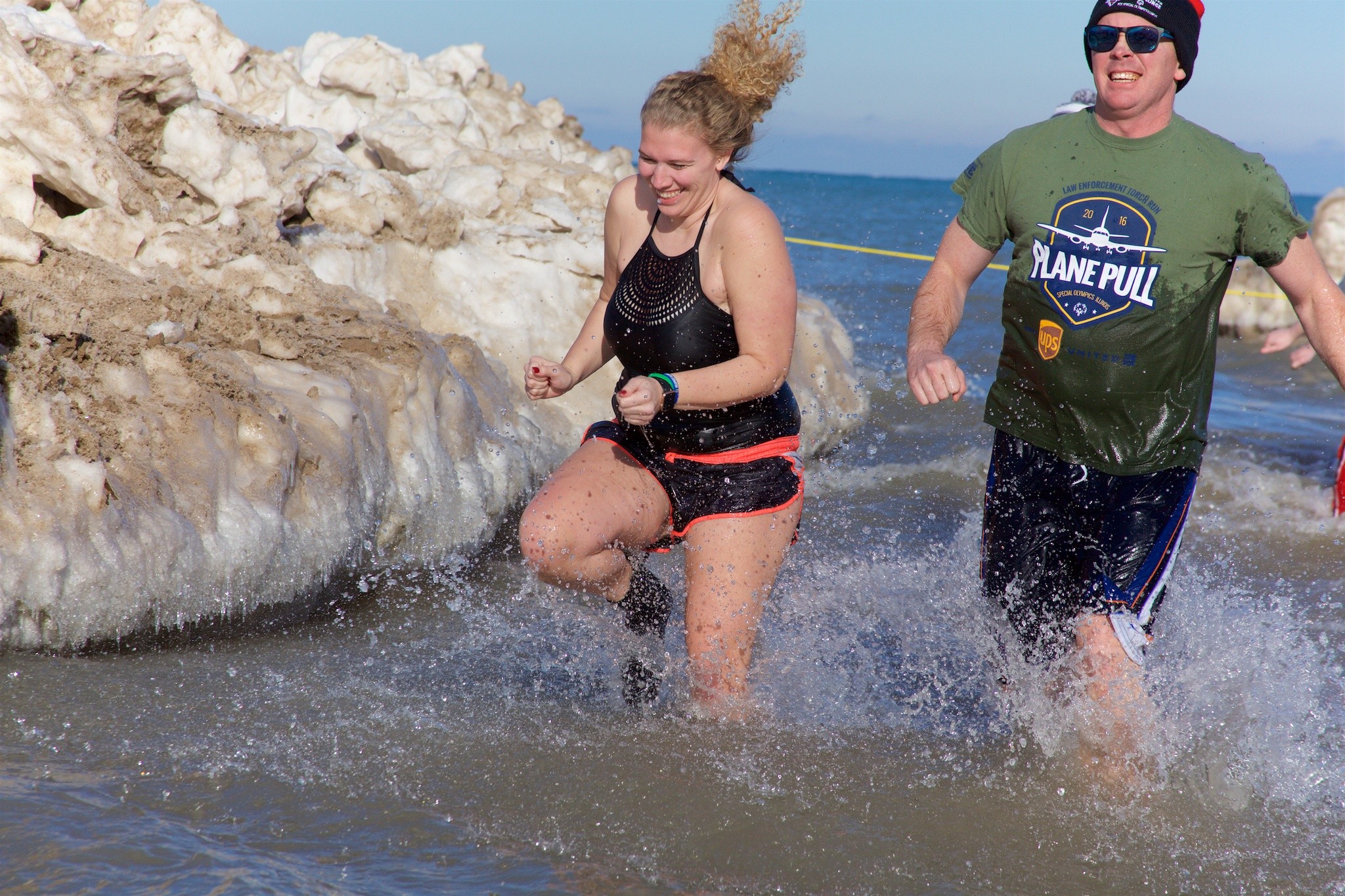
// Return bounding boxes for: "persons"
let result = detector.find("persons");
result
[1259,275,1344,370]
[907,0,1343,782]
[518,0,812,721]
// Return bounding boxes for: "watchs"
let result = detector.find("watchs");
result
[649,372,677,413]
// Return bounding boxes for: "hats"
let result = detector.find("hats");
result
[1084,0,1205,94]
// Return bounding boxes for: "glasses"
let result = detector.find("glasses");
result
[1084,25,1174,53]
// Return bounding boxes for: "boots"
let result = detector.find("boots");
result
[606,551,675,707]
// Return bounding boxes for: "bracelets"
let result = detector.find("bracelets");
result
[649,372,680,407]
